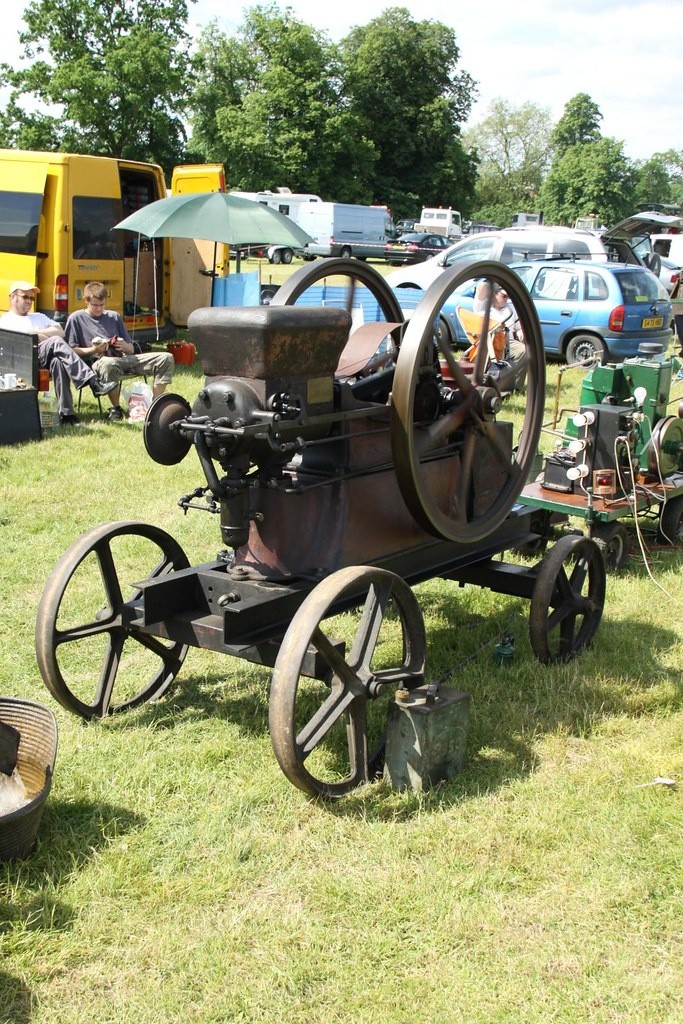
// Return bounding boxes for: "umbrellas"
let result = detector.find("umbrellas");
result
[110,193,317,307]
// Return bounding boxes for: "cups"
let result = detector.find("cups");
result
[3,373,17,389]
[0,376,6,391]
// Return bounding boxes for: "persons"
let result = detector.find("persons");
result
[0,281,118,429]
[64,282,175,420]
[475,282,529,392]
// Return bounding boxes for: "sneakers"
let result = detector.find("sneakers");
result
[58,413,81,427]
[92,378,117,398]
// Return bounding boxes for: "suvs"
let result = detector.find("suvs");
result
[384,210,683,298]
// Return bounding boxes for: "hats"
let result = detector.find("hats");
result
[9,281,40,295]
[492,282,507,296]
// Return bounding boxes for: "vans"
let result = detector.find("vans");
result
[649,234,683,269]
[0,148,230,346]
[297,203,402,261]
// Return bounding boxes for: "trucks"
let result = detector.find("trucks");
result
[421,208,463,239]
[511,210,544,227]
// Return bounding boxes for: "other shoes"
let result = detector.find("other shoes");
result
[108,406,124,422]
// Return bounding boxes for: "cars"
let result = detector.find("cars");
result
[385,233,455,266]
[612,252,682,294]
[432,258,674,370]
[396,218,419,231]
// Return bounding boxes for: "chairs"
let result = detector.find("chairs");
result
[77,340,146,418]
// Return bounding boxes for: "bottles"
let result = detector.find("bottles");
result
[494,635,515,666]
[39,391,60,434]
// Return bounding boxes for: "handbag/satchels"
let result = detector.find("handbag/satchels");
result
[122,381,153,424]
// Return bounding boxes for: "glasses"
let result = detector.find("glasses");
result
[502,295,507,298]
[17,294,36,301]
